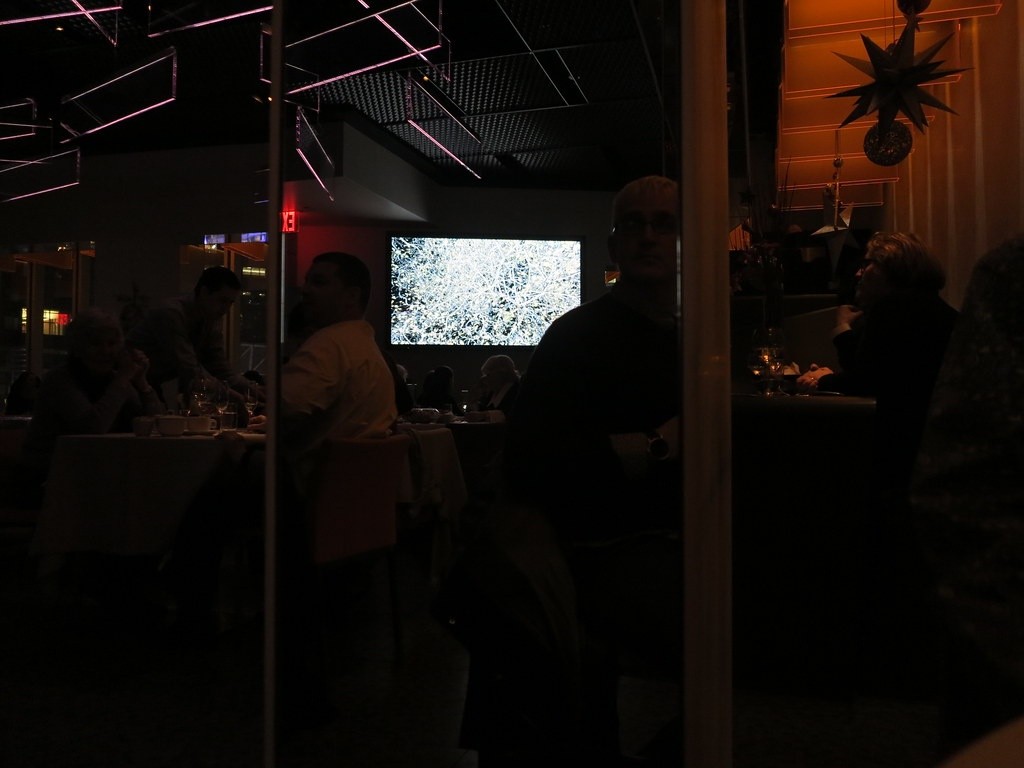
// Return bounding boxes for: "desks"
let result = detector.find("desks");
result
[386,414,513,501]
[685,390,903,666]
[58,430,273,590]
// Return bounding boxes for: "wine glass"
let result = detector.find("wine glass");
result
[243,380,259,434]
[750,327,791,400]
[456,384,473,417]
[213,379,230,436]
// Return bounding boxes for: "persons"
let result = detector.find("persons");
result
[287,301,523,433]
[6,264,269,509]
[732,225,959,461]
[278,252,399,496]
[502,179,746,767]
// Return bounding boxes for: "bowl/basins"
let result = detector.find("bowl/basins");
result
[409,407,440,424]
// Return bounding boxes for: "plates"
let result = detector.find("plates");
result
[184,430,217,435]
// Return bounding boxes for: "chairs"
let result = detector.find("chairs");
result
[319,429,483,667]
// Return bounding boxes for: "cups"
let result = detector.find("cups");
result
[156,415,185,436]
[222,412,239,432]
[132,416,153,437]
[406,383,421,409]
[187,416,217,431]
